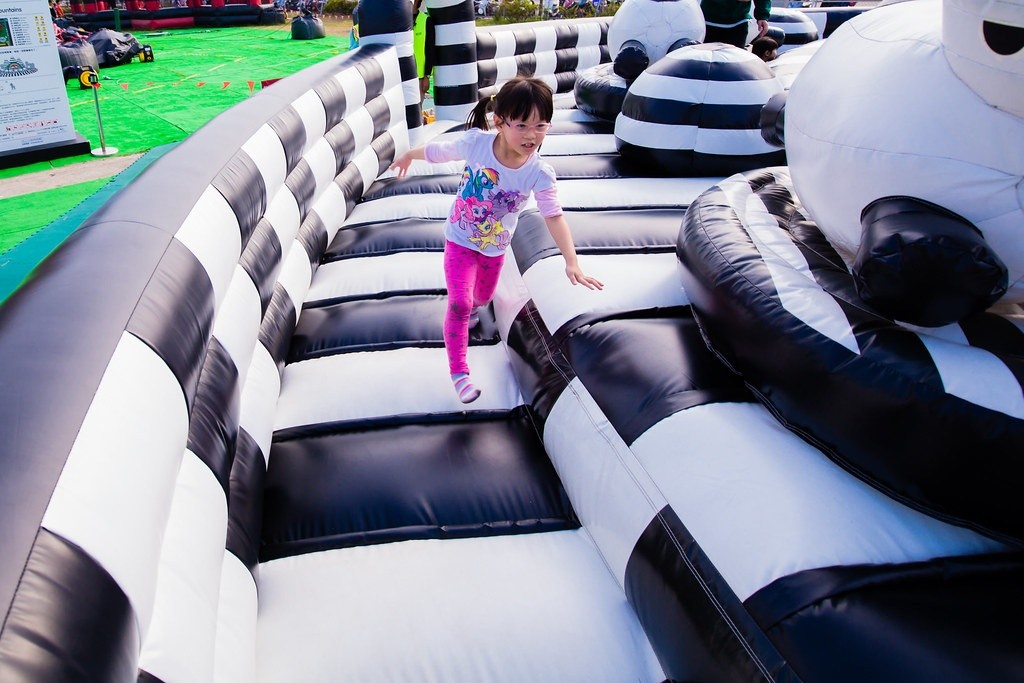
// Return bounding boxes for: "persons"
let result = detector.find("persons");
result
[273,0,321,20]
[412,0,436,108]
[701,0,772,51]
[388,78,604,404]
[752,37,778,62]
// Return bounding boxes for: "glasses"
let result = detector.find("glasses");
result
[501,116,552,134]
